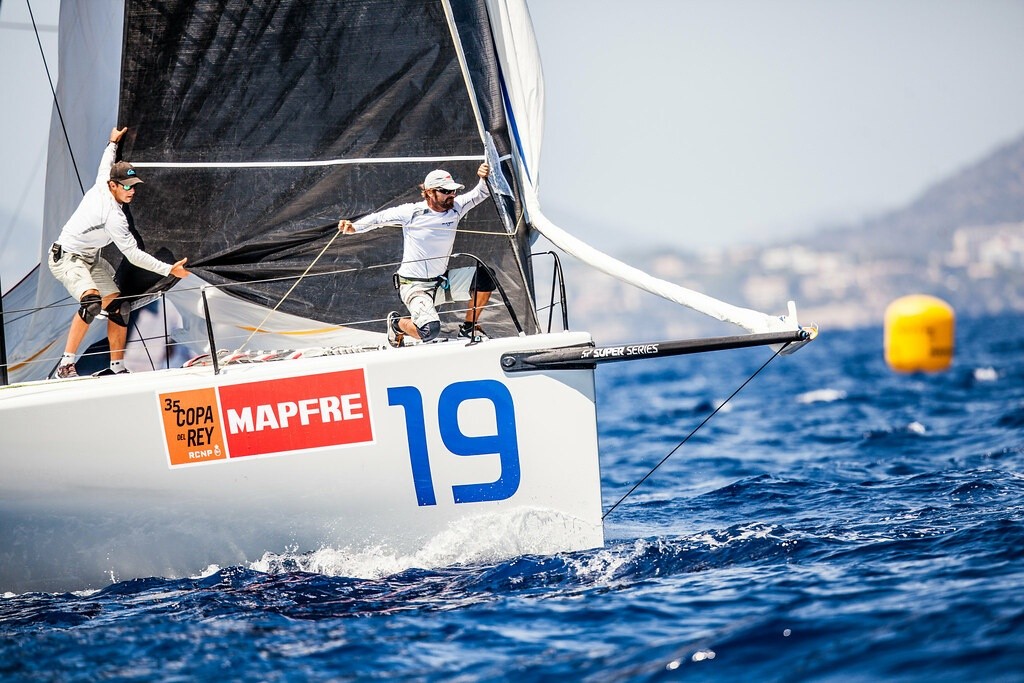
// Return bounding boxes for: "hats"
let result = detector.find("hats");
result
[110,160,144,186]
[422,169,465,191]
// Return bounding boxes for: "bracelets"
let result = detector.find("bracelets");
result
[107,140,118,145]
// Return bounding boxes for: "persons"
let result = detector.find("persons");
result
[46,127,190,379]
[337,162,496,348]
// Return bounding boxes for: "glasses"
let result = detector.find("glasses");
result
[435,187,457,195]
[113,181,137,190]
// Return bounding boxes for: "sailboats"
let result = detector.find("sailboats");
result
[0,0,819,596]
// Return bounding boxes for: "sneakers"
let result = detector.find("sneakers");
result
[456,324,494,340]
[386,311,405,348]
[54,362,79,378]
